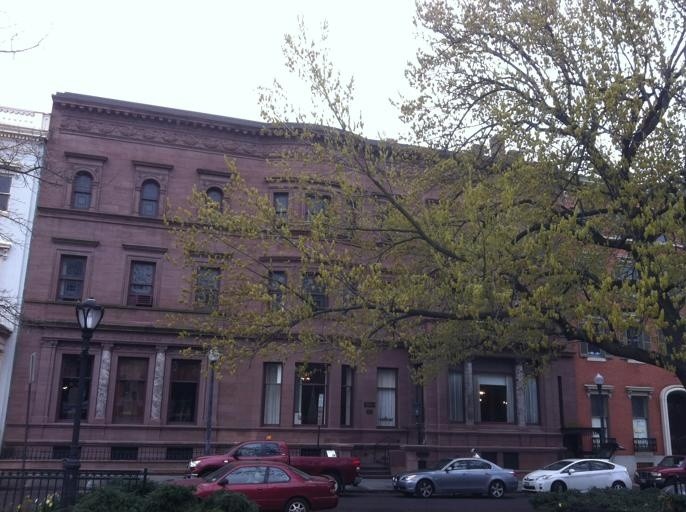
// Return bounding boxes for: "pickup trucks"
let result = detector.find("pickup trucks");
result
[185,439,363,498]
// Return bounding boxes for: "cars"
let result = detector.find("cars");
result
[390,457,519,500]
[520,457,633,495]
[162,459,340,512]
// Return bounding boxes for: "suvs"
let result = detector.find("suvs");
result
[633,453,685,492]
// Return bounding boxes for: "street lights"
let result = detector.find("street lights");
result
[594,372,609,460]
[58,294,107,511]
[203,346,219,455]
